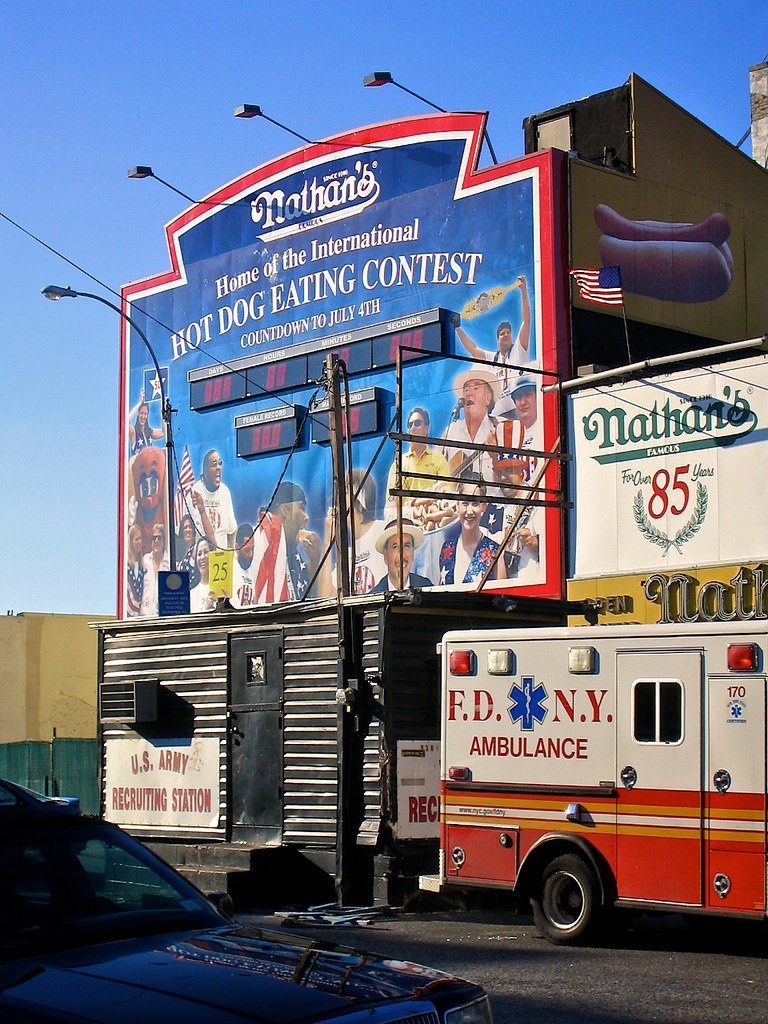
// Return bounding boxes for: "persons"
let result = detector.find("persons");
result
[125,276,545,586]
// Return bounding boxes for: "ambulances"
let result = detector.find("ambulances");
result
[436,620,768,941]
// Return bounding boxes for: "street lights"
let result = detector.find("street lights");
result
[41,282,175,570]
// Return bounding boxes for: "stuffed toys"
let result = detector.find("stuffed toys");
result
[127,445,166,555]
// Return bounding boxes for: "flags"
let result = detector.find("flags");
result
[254,504,314,603]
[569,264,624,307]
[174,445,196,527]
[127,562,143,618]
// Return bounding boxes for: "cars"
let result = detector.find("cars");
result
[0,813,494,1023]
[0,779,82,821]
